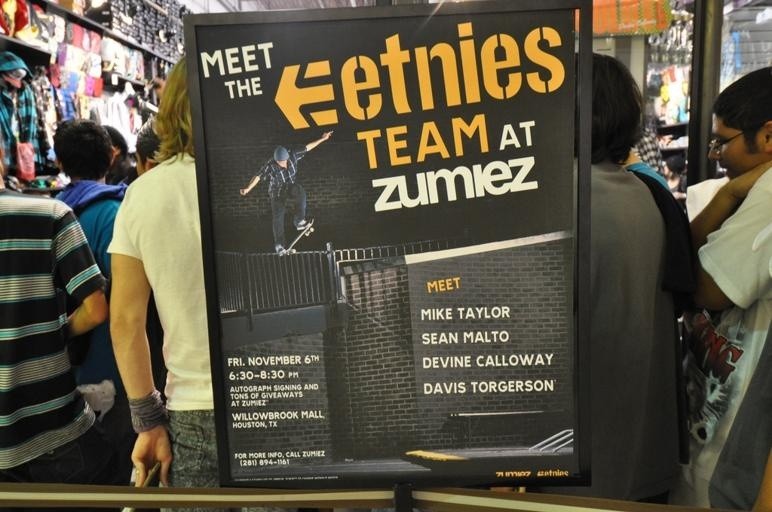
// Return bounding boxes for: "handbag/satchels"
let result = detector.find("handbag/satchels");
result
[16,141,35,180]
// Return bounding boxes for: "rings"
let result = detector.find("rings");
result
[136,469,140,474]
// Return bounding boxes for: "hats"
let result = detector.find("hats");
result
[2,69,26,89]
[273,146,290,161]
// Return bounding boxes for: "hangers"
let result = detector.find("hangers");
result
[117,72,160,115]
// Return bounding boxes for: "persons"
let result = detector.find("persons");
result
[661,150,685,194]
[109,55,298,512]
[236,127,334,253]
[538,50,696,504]
[103,123,131,183]
[685,64,772,512]
[50,117,170,487]
[137,128,164,180]
[0,142,109,482]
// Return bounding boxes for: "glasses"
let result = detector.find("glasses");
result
[708,130,743,154]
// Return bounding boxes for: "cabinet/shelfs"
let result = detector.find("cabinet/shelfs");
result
[0,0,183,87]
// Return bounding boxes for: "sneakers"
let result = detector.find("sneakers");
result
[296,219,312,230]
[275,245,286,254]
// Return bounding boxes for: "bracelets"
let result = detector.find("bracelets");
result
[127,391,169,434]
